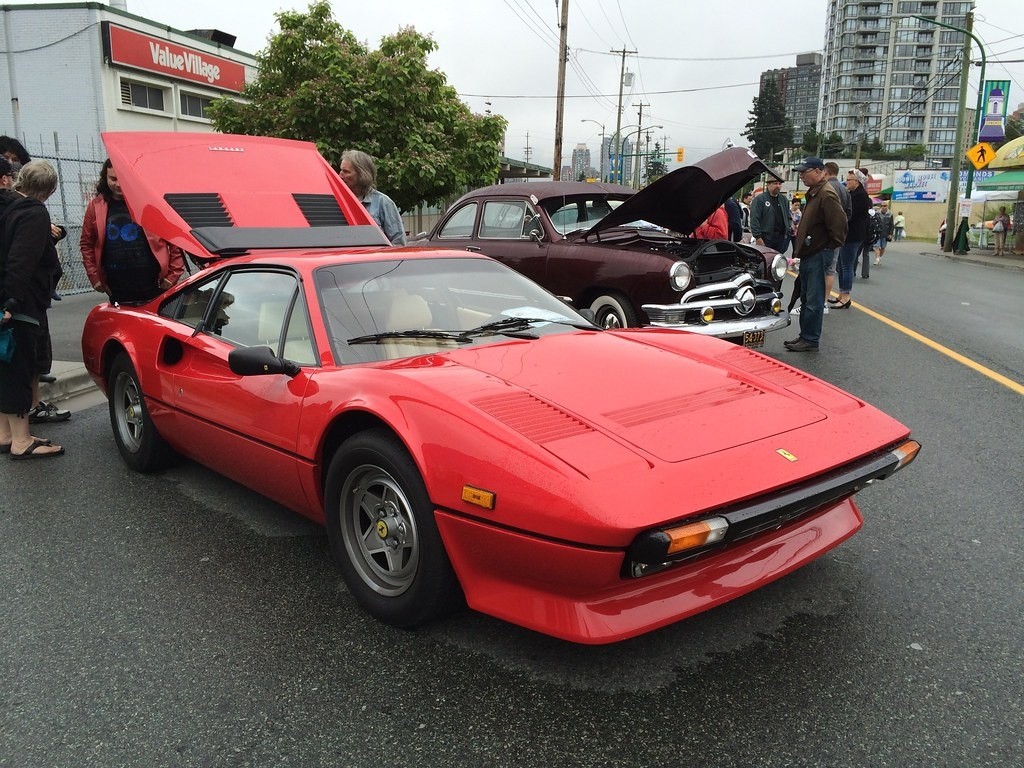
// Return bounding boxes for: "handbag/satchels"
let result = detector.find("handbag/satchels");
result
[940,219,947,230]
[901,230,906,237]
[993,221,1004,233]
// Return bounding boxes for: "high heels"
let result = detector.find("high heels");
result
[828,298,840,303]
[830,300,851,309]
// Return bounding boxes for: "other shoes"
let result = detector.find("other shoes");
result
[40,374,57,382]
[28,400,71,423]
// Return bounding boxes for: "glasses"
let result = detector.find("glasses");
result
[847,179,854,181]
[6,173,16,179]
[881,205,886,207]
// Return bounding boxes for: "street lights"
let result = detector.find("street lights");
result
[581,120,605,182]
[888,13,986,254]
[622,125,663,185]
[794,126,825,161]
[608,125,643,157]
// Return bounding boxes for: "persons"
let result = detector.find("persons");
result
[748,173,794,299]
[339,150,408,291]
[939,219,948,250]
[784,157,848,352]
[873,201,894,265]
[892,212,905,242]
[992,206,1010,256]
[690,162,880,314]
[0,136,71,459]
[79,158,187,305]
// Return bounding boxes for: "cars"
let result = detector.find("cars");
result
[81,131,921,645]
[409,148,792,349]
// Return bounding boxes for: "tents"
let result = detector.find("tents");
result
[954,189,1024,247]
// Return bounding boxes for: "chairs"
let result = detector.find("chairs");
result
[256,296,316,364]
[378,294,458,359]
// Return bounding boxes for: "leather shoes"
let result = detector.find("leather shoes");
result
[784,337,820,350]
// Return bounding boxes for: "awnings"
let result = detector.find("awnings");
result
[976,168,1024,192]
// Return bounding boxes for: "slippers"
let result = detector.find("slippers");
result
[0,434,65,459]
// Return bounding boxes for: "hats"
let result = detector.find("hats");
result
[853,169,867,186]
[792,156,824,172]
[881,201,888,205]
[766,174,785,183]
